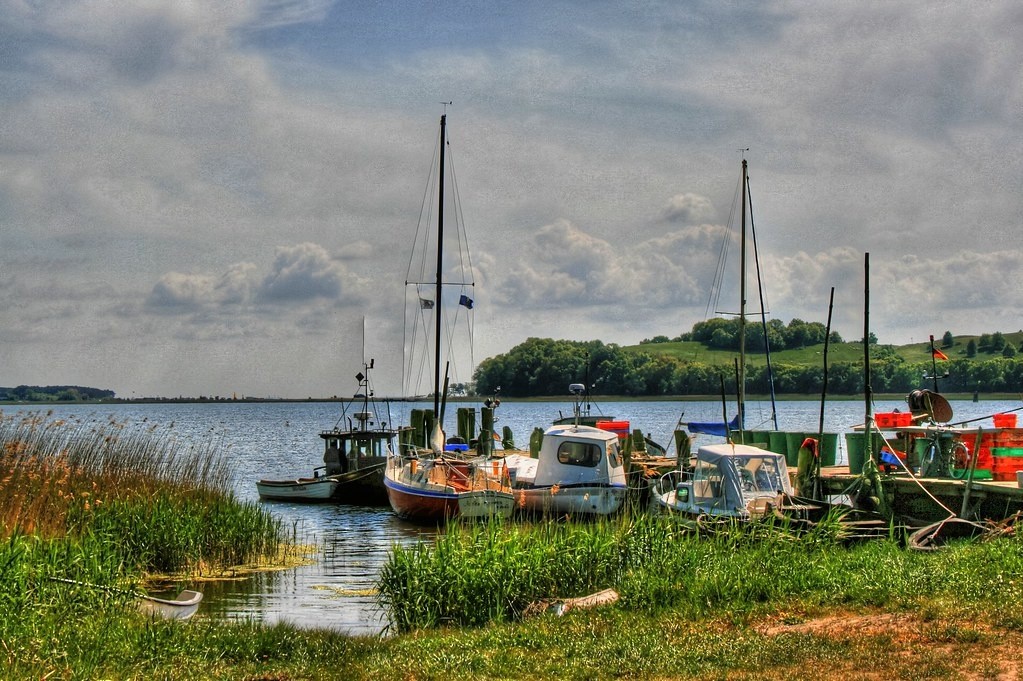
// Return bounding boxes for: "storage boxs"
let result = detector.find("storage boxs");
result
[597,420,630,438]
[874,412,912,428]
[878,447,907,471]
[955,414,1023,481]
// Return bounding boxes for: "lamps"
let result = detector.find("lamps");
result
[484,398,492,408]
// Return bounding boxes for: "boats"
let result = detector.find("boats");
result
[252,315,398,505]
[48,575,204,625]
[650,442,833,539]
[478,98,1023,549]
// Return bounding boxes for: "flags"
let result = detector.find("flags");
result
[420,298,434,309]
[459,295,474,310]
[932,347,948,360]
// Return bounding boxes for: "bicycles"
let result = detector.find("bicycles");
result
[918,425,971,480]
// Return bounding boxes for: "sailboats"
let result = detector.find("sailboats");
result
[381,93,519,526]
